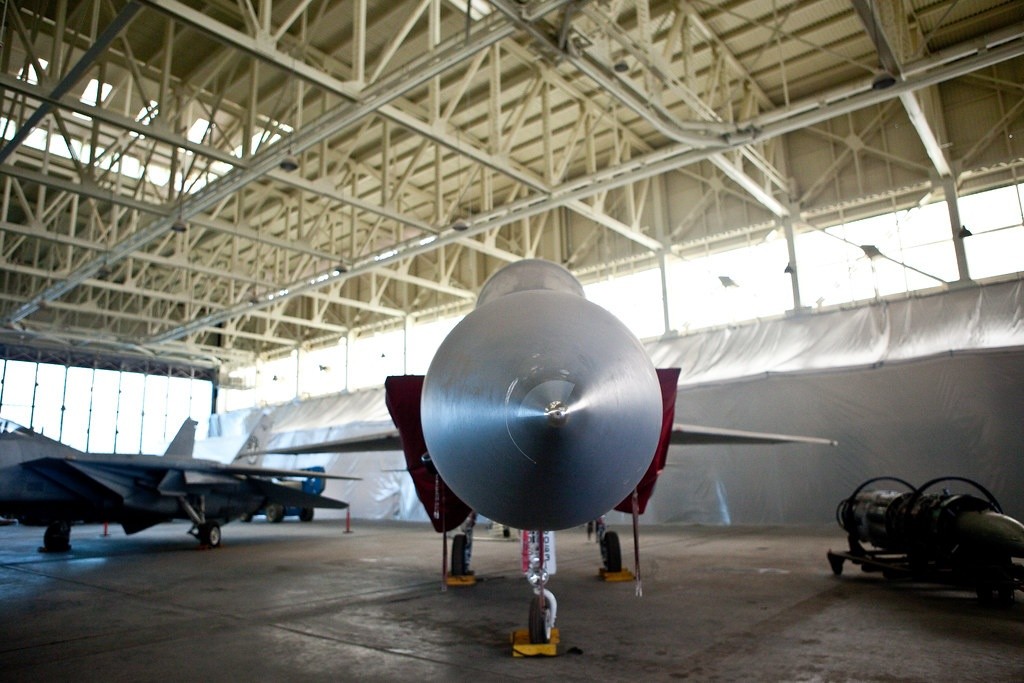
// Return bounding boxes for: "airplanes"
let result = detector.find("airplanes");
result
[0,413,364,554]
[233,265,841,655]
